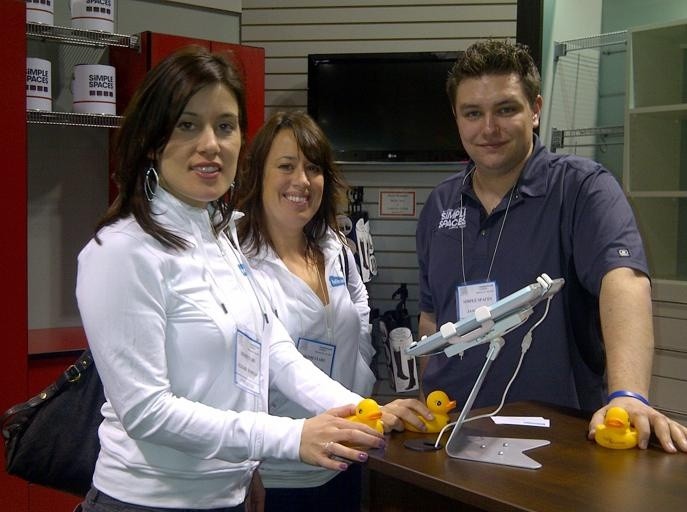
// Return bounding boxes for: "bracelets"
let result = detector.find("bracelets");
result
[607,391,650,405]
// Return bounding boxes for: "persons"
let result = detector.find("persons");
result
[411,36,686,459]
[74,44,390,507]
[230,107,379,507]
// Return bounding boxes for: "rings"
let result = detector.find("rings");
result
[325,440,334,456]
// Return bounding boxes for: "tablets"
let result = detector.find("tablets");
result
[405,279,564,357]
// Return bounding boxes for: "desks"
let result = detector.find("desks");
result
[344,402,685,512]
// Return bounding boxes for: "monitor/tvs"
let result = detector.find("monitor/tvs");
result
[307,52,465,164]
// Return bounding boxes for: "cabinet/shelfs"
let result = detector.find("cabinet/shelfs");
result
[109,31,265,209]
[25,25,141,136]
[619,19,685,283]
[646,275,685,412]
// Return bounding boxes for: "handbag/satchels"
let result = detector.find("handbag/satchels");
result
[0,347,106,497]
